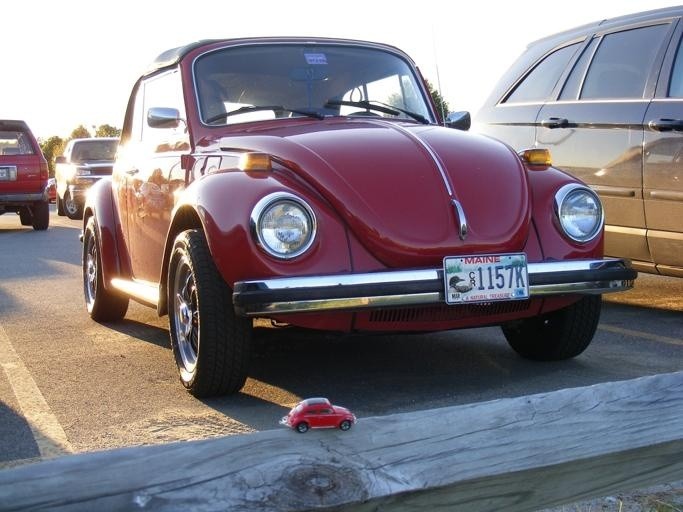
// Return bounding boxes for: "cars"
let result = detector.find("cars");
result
[45,177,58,202]
[75,34,637,400]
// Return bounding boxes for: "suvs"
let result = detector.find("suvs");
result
[465,7,682,316]
[0,119,50,231]
[53,137,120,222]
